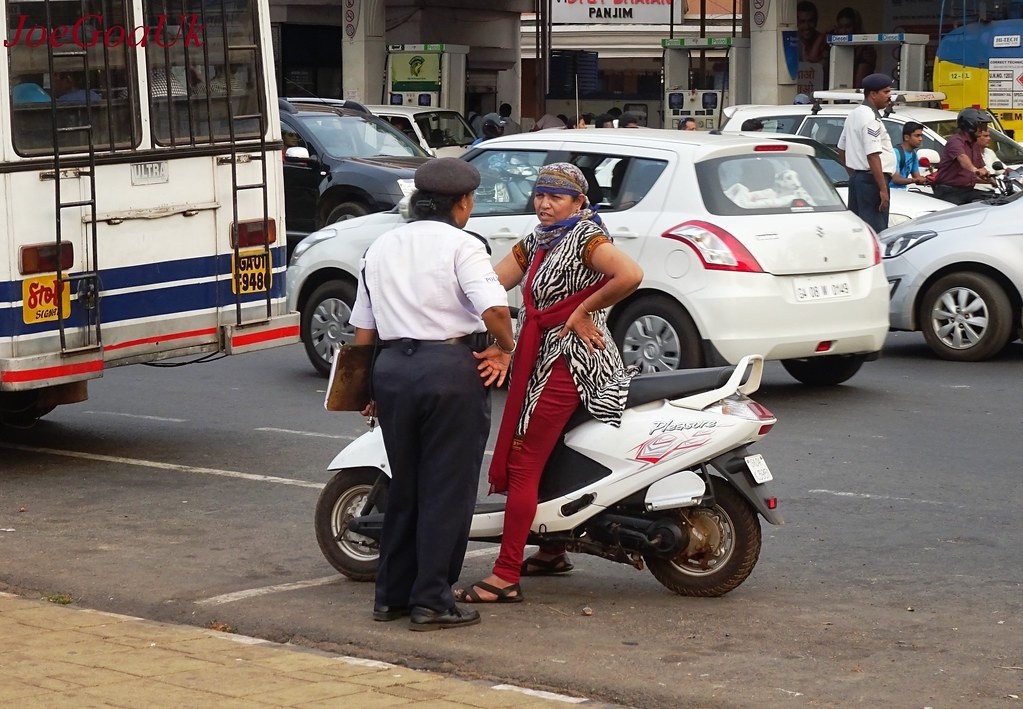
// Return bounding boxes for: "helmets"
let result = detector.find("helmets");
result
[957,108,993,133]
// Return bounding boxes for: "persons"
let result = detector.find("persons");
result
[498,103,521,136]
[888,122,926,190]
[534,114,568,131]
[348,157,515,632]
[13,71,102,104]
[931,107,996,206]
[678,118,696,131]
[117,65,245,100]
[453,162,644,604]
[837,73,897,233]
[567,107,637,128]
[281,122,298,163]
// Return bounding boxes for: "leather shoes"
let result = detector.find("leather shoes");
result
[409,605,481,631]
[373,602,411,621]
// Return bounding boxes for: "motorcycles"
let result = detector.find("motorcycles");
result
[975,160,1023,196]
[915,157,939,192]
[314,306,783,599]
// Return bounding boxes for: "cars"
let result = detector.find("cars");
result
[595,129,959,227]
[719,88,1023,194]
[877,192,1023,360]
[287,128,890,390]
[358,105,522,167]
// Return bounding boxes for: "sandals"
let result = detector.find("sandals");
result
[520,553,574,576]
[456,581,524,603]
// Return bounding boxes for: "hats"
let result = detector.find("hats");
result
[793,94,812,105]
[862,73,891,90]
[414,157,481,195]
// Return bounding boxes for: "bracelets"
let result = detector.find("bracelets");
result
[494,338,517,354]
[581,304,594,315]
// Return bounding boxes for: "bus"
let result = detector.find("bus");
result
[2,1,301,428]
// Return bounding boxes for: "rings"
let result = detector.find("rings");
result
[593,333,599,341]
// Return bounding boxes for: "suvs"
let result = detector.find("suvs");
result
[278,97,535,274]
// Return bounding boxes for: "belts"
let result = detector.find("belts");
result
[854,169,892,180]
[382,337,471,345]
